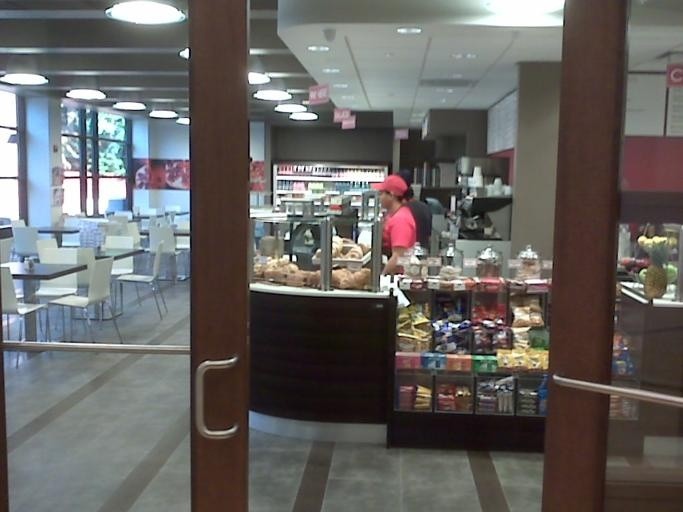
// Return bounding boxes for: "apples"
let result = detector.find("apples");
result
[662,264,678,283]
[638,235,678,248]
[639,268,647,282]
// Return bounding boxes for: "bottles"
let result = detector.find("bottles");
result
[516,243,542,279]
[477,244,504,276]
[24,258,33,270]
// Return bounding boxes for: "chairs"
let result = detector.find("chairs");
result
[1,205,190,369]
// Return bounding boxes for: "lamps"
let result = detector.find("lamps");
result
[0,0,319,124]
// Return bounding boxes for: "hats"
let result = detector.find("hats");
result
[372,176,408,197]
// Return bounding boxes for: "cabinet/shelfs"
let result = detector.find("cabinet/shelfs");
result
[249,205,683,456]
[273,161,389,214]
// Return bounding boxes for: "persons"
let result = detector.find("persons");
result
[402,186,432,248]
[370,175,417,275]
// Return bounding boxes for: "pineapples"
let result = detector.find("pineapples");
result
[644,240,667,300]
